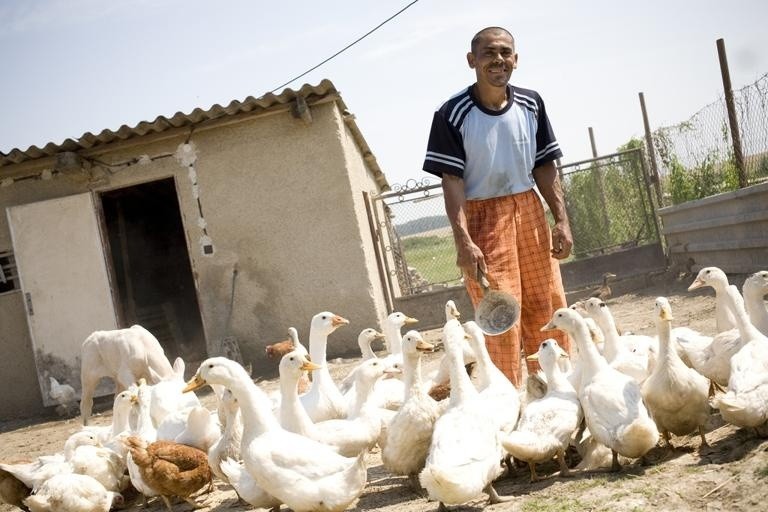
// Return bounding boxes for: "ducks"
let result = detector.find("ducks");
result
[0,265,768,512]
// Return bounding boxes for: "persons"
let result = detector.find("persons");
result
[422,26,574,389]
